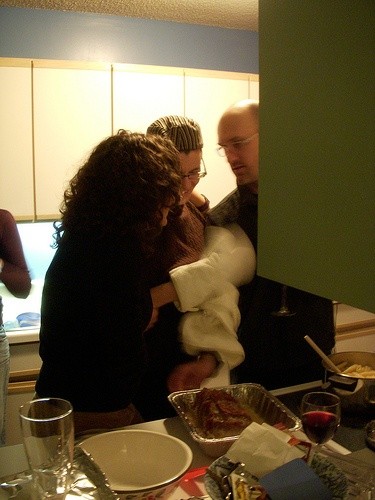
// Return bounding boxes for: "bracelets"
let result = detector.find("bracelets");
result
[196,350,225,378]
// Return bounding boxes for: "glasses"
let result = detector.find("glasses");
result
[216,133,259,157]
[182,156,207,179]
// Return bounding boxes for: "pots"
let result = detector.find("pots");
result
[322,351,375,426]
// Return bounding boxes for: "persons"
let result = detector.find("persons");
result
[26,129,257,434]
[147,116,217,272]
[0,210,32,447]
[159,96,337,417]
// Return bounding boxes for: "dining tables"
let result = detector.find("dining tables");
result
[0,381,375,500]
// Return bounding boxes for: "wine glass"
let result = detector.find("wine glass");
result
[271,285,295,316]
[301,393,340,446]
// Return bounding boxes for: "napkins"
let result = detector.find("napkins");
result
[225,422,306,478]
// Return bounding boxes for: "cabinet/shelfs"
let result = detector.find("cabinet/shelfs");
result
[257,0,375,313]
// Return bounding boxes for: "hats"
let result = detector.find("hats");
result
[146,115,203,150]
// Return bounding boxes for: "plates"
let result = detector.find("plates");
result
[204,454,347,500]
[167,383,302,457]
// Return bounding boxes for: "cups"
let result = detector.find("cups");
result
[18,398,73,500]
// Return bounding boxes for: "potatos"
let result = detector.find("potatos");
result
[330,361,375,378]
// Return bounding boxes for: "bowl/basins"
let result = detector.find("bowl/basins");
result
[73,430,192,500]
